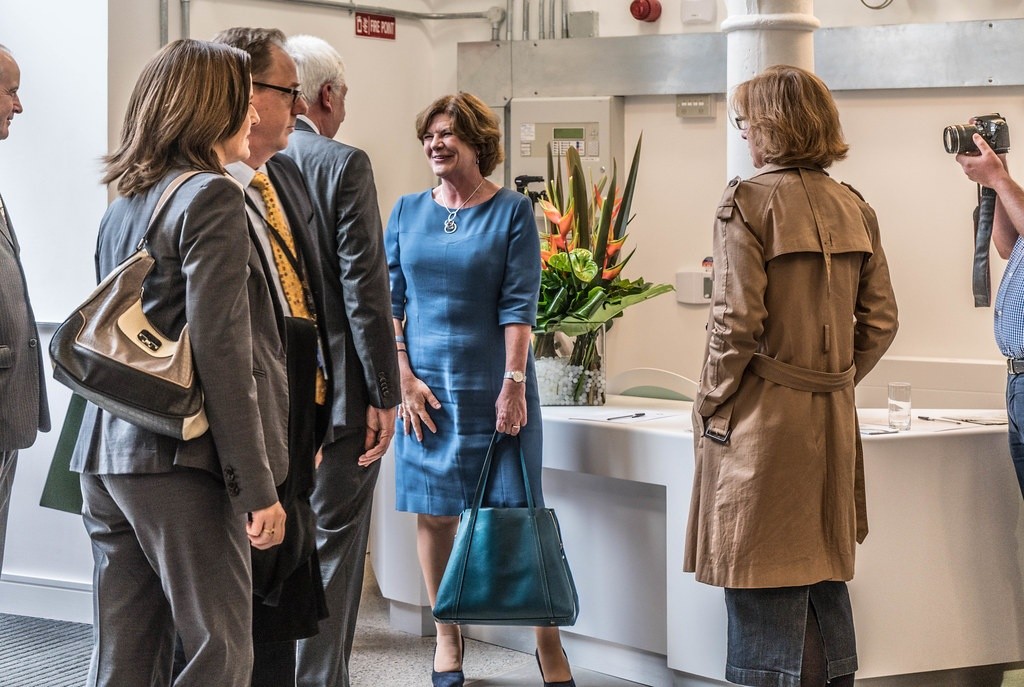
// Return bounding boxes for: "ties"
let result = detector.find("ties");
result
[248,168,329,410]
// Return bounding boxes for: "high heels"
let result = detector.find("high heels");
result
[535,645,576,687]
[431,633,465,687]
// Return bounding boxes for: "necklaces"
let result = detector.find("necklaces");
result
[441,179,484,234]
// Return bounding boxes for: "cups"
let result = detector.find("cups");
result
[888,381,912,431]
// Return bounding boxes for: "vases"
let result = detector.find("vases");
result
[531,322,607,406]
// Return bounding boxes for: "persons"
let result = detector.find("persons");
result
[955,133,1024,496]
[280,34,403,687]
[70,38,290,687]
[212,27,335,687]
[683,65,899,687]
[0,44,52,574]
[384,93,576,687]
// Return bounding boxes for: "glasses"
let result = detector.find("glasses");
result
[249,80,302,118]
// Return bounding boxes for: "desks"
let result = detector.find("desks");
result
[368,394,1024,686]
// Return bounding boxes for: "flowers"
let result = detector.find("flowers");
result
[526,127,676,405]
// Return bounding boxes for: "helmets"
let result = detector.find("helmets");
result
[730,113,749,132]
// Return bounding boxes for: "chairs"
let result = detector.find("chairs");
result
[605,367,700,403]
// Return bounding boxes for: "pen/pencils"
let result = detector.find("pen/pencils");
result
[918,416,962,425]
[607,413,645,420]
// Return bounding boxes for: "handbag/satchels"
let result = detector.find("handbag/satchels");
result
[427,428,577,626]
[47,170,213,444]
[279,308,319,505]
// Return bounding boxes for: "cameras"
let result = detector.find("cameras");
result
[944,113,1011,155]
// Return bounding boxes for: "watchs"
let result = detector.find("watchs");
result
[503,371,527,383]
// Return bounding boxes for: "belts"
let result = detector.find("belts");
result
[1006,358,1024,375]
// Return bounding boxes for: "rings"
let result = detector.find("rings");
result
[263,528,275,536]
[513,425,520,429]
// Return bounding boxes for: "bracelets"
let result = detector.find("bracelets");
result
[395,336,405,342]
[397,348,406,353]
